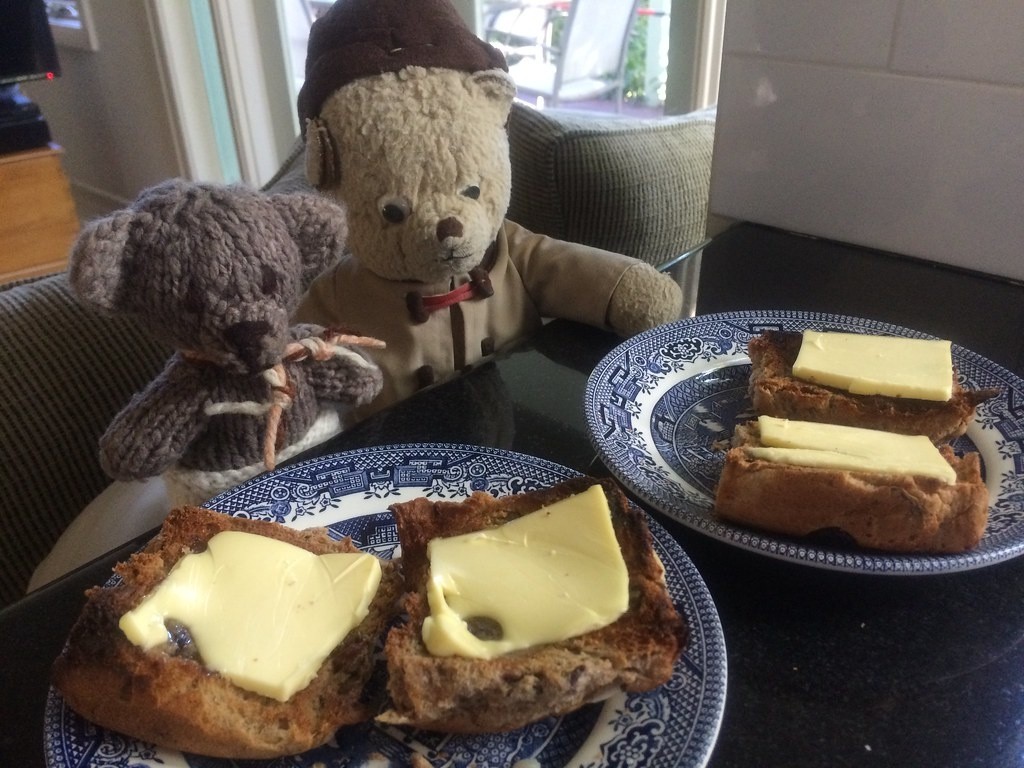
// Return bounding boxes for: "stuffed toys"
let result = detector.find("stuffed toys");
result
[293,66,683,426]
[295,1,507,143]
[70,177,384,516]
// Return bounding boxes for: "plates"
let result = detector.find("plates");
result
[46,442,729,768]
[583,310,1024,573]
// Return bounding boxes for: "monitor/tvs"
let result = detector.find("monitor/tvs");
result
[0,0,62,88]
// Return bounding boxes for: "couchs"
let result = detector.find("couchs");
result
[0,103,716,614]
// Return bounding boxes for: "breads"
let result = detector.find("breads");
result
[51,507,388,760]
[376,478,676,732]
[754,335,997,436]
[716,423,991,552]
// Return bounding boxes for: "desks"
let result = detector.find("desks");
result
[0,221,1024,768]
